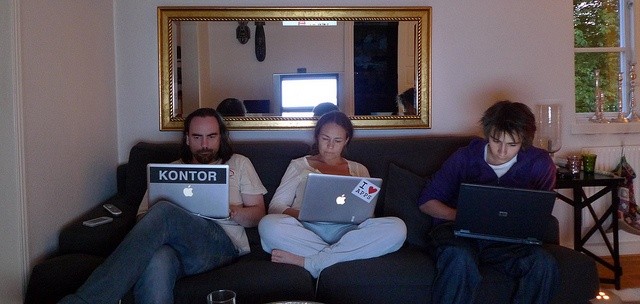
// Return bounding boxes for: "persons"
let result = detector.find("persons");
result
[57,108,268,304]
[217,98,247,117]
[312,102,339,116]
[398,87,416,114]
[417,100,560,304]
[258,110,407,279]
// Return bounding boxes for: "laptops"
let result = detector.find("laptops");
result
[146,162,229,220]
[243,99,272,114]
[362,109,397,116]
[452,183,556,246]
[297,173,382,225]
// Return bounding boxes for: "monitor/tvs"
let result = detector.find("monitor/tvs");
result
[270,73,343,111]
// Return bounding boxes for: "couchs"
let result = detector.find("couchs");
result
[23,136,600,303]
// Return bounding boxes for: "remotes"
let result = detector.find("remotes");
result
[103,203,122,216]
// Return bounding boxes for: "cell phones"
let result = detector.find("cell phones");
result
[83,216,113,227]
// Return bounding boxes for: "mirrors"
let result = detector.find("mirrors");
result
[156,5,433,132]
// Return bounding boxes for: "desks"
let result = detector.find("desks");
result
[554,169,624,291]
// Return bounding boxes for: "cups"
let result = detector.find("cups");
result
[207,289,238,303]
[585,154,596,173]
[568,156,582,174]
